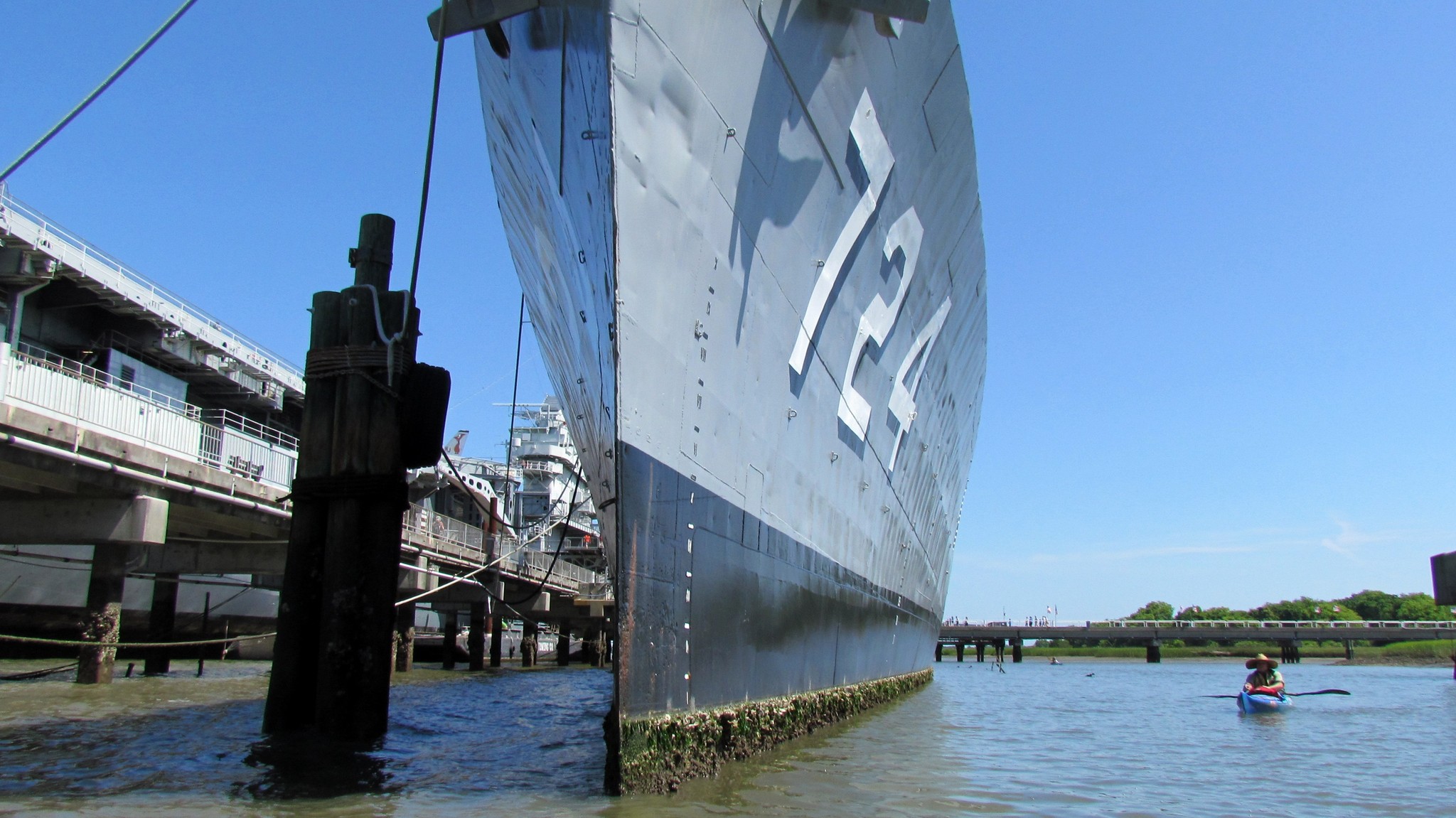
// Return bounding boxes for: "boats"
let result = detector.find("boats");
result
[1237,691,1293,714]
[1050,662,1063,665]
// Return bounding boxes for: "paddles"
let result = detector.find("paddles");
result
[1190,688,1351,698]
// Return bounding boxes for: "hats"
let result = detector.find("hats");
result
[1246,653,1277,669]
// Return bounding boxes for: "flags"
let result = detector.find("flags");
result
[1046,606,1058,614]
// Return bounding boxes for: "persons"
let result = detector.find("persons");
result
[584,534,591,549]
[1050,657,1059,664]
[1243,653,1286,697]
[945,616,1050,627]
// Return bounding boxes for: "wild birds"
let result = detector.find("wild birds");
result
[1087,673,1095,677]
